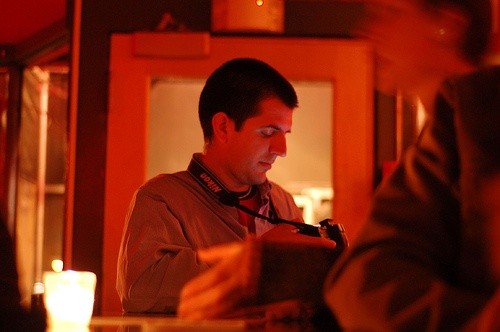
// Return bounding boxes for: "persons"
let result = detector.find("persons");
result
[324,62,500,332]
[117,58,337,317]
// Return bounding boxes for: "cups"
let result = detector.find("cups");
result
[43,269,97,332]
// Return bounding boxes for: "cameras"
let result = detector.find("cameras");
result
[298,218,349,268]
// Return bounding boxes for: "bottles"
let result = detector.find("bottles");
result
[26,282,47,331]
